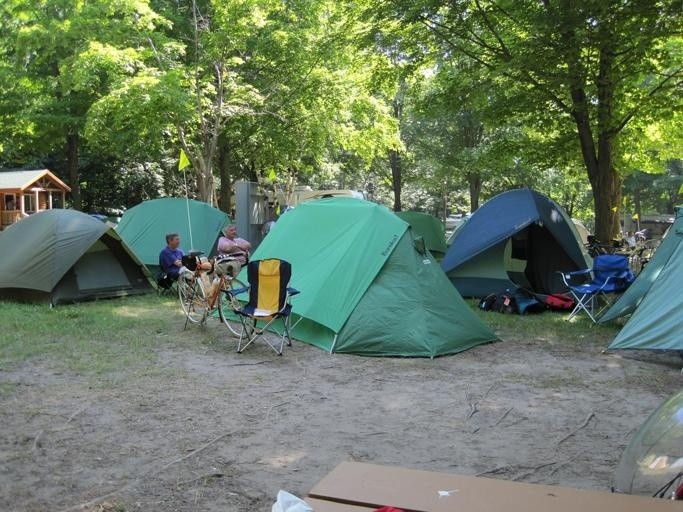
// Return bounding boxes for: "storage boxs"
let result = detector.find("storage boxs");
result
[303,461,683,512]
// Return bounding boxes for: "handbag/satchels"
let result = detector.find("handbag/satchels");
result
[478,292,497,311]
[493,291,514,315]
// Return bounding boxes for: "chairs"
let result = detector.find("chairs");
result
[161,278,182,298]
[229,258,300,355]
[561,255,636,324]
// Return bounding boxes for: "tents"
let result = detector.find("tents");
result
[0,208,158,307]
[112,195,232,269]
[228,196,504,359]
[436,186,599,310]
[592,205,682,369]
[393,210,448,267]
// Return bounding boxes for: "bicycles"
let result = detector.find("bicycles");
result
[586,233,655,278]
[177,250,256,339]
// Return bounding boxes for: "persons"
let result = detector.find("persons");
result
[157,232,188,280]
[214,224,252,267]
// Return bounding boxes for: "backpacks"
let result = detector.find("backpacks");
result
[514,287,544,316]
